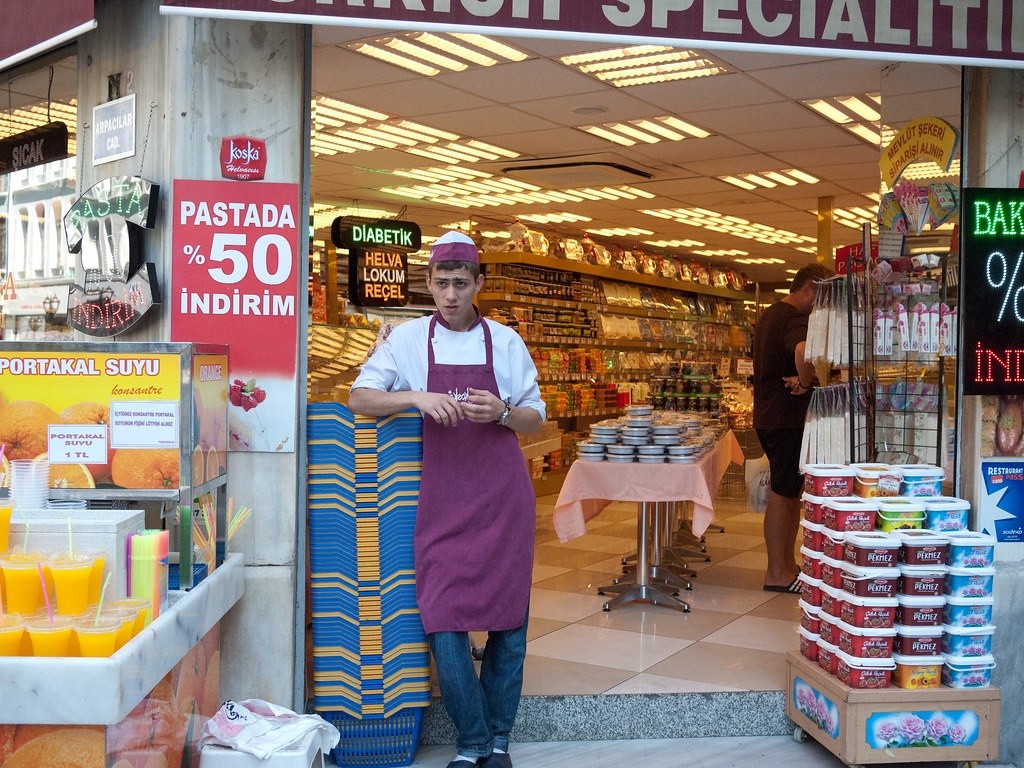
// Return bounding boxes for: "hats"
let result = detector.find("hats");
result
[429,228,479,263]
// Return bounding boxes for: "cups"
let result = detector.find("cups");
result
[0,498,12,555]
[10,459,87,511]
[0,551,149,659]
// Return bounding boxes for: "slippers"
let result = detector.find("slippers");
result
[763,575,803,594]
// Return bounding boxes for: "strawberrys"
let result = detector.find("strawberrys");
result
[230,378,267,413]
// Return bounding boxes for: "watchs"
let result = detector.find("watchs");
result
[497,401,513,426]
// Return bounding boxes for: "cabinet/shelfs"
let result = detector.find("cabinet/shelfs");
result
[472,250,751,419]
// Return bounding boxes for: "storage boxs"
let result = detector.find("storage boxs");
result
[785,649,1002,768]
[8,509,145,602]
[796,462,997,687]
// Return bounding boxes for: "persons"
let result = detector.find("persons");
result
[751,262,834,593]
[348,231,546,768]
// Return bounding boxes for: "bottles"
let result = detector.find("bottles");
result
[609,241,623,268]
[688,258,700,282]
[631,245,644,269]
[509,219,525,252]
[670,255,680,279]
[651,250,664,277]
[579,234,594,263]
[546,228,562,259]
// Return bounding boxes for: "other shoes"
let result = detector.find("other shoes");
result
[478,752,513,768]
[446,759,476,768]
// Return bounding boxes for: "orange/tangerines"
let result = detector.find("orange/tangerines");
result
[0,401,180,490]
[191,446,220,487]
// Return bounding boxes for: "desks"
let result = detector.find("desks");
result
[550,426,746,614]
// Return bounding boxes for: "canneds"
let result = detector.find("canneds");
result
[501,262,599,337]
[645,378,721,413]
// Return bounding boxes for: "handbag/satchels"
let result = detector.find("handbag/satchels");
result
[200,697,341,760]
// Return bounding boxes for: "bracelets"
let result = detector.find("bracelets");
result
[798,376,810,389]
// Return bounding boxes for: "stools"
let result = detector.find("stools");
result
[199,729,325,768]
